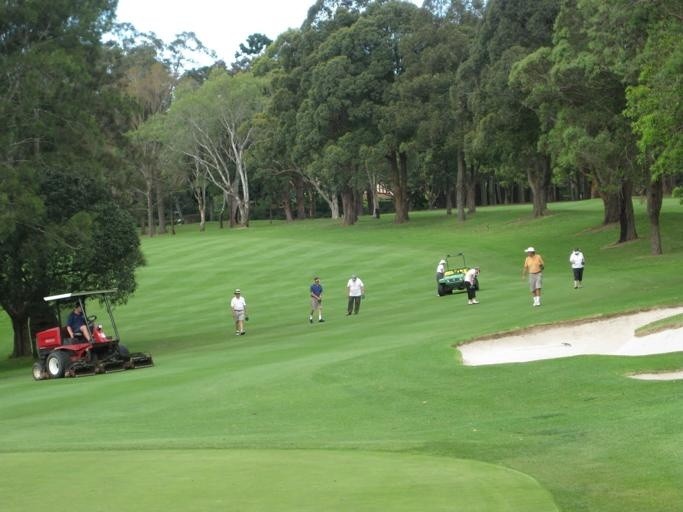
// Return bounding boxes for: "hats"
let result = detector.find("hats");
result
[524,246,535,252]
[232,289,240,294]
[314,276,320,282]
[351,274,356,281]
[438,259,447,264]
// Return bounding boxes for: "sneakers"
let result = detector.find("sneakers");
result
[234,329,246,336]
[309,318,325,323]
[468,300,480,305]
[573,285,583,289]
[532,301,541,307]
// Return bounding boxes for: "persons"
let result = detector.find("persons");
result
[520,247,544,308]
[307,277,324,323]
[435,259,446,296]
[230,288,247,336]
[464,267,480,305]
[343,274,365,316]
[66,302,94,343]
[568,248,585,289]
